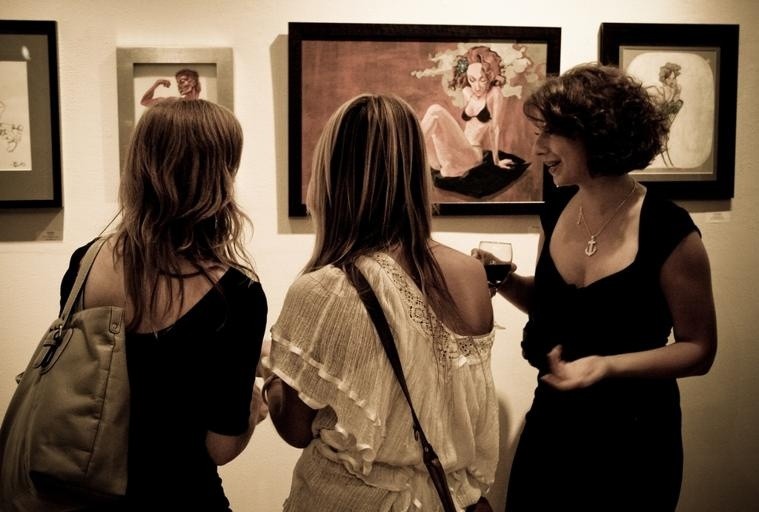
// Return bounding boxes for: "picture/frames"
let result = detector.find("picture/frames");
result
[287,19,564,220]
[1,19,67,215]
[597,20,743,205]
[114,46,236,198]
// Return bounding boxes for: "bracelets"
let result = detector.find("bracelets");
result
[261,373,279,406]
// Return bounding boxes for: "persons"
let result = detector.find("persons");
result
[420,45,515,177]
[140,70,202,107]
[640,64,683,167]
[470,63,717,511]
[259,94,501,512]
[59,101,270,511]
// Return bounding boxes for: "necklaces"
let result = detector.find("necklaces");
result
[576,178,637,256]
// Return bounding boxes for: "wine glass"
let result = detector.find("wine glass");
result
[477,241,514,330]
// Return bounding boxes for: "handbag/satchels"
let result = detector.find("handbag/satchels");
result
[0,301,131,498]
